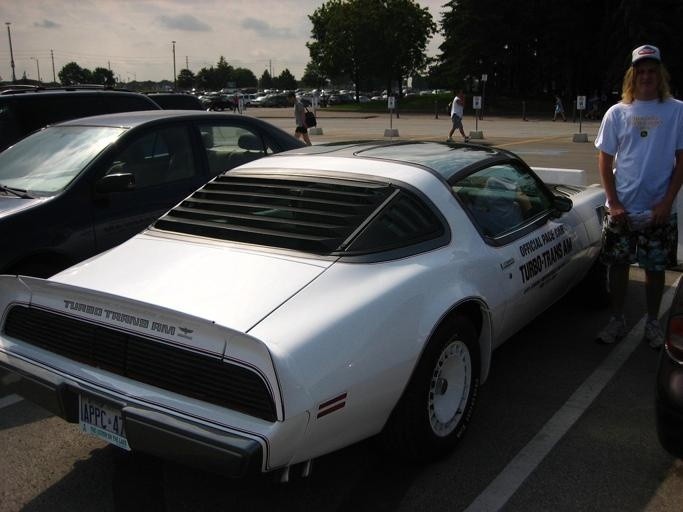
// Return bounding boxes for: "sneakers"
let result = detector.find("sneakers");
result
[598,314,627,343]
[644,319,665,349]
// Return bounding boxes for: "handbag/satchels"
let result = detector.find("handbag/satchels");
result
[305,110,316,128]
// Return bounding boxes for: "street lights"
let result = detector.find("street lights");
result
[172,41,177,90]
[29,57,41,81]
[4,21,17,81]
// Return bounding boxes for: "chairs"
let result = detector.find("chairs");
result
[105,131,269,190]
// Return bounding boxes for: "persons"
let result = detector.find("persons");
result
[594,44,682,350]
[447,89,470,142]
[287,91,311,146]
[584,88,599,120]
[552,94,567,121]
[232,95,244,115]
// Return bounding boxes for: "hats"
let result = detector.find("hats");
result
[631,45,661,66]
